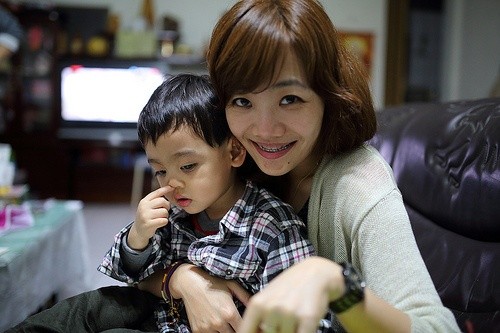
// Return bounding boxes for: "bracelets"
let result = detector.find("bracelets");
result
[161,259,192,326]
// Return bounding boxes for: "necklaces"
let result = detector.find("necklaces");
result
[289,161,319,206]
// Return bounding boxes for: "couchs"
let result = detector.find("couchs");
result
[364,97,500,333]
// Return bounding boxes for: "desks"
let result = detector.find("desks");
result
[0,199,88,333]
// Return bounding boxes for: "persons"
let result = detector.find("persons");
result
[2,73,344,333]
[0,9,22,64]
[136,0,462,333]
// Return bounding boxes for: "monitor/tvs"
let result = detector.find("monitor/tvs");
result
[53,56,168,129]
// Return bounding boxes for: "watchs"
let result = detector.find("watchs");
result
[328,262,365,315]
